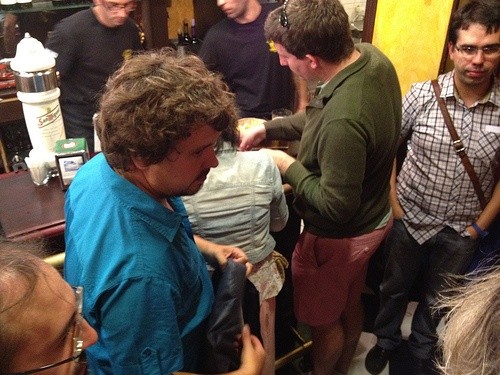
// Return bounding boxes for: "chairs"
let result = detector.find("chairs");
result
[0,98,24,174]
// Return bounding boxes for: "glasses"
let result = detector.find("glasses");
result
[8,284,85,375]
[99,0,137,13]
[453,44,500,59]
[279,0,289,33]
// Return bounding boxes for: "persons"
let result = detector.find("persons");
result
[44,0,145,151]
[178,95,290,306]
[241,0,407,374]
[62,44,270,374]
[363,0,500,374]
[194,0,298,125]
[2,236,99,374]
[428,249,499,374]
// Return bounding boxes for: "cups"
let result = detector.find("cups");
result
[26,157,51,186]
[271,108,294,149]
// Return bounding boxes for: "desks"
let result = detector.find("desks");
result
[0,139,302,241]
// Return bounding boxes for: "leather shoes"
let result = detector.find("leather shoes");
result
[365,344,391,375]
